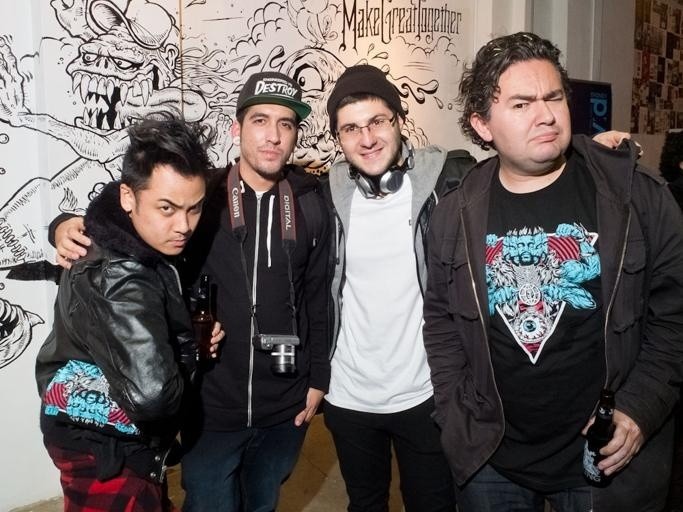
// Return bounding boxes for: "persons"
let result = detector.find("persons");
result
[311,65,644,511]
[46,71,334,511]
[35,114,226,512]
[421,31,680,511]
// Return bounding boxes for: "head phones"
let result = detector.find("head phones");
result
[347,134,416,198]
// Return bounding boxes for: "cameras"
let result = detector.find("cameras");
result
[253,333,301,375]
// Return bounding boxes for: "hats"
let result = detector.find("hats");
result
[326,64,404,114]
[235,72,311,124]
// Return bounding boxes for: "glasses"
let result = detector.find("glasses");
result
[333,111,396,140]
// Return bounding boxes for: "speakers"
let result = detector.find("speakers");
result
[566,78,613,140]
